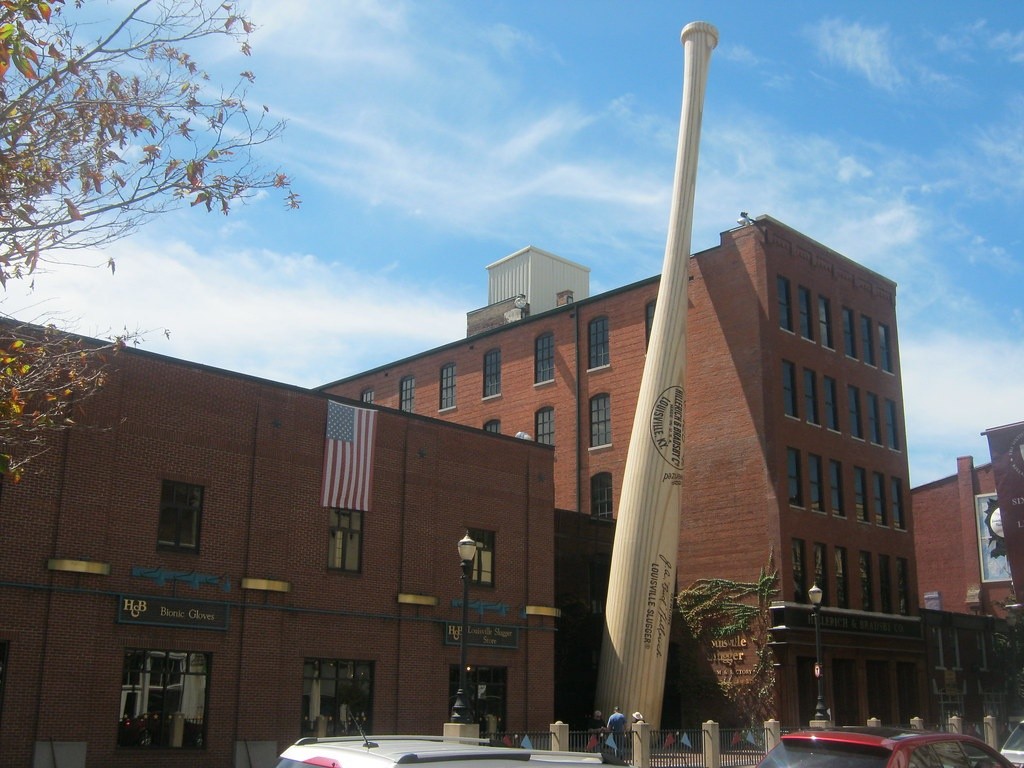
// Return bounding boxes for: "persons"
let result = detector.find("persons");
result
[588,710,606,742]
[605,706,627,760]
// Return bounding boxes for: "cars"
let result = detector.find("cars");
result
[754,727,1018,768]
[996,720,1024,768]
[302,694,367,729]
[118,713,204,749]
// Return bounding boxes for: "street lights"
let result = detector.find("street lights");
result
[450,529,476,723]
[808,581,830,720]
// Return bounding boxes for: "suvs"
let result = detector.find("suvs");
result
[271,708,638,768]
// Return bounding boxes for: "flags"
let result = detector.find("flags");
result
[319,399,378,512]
